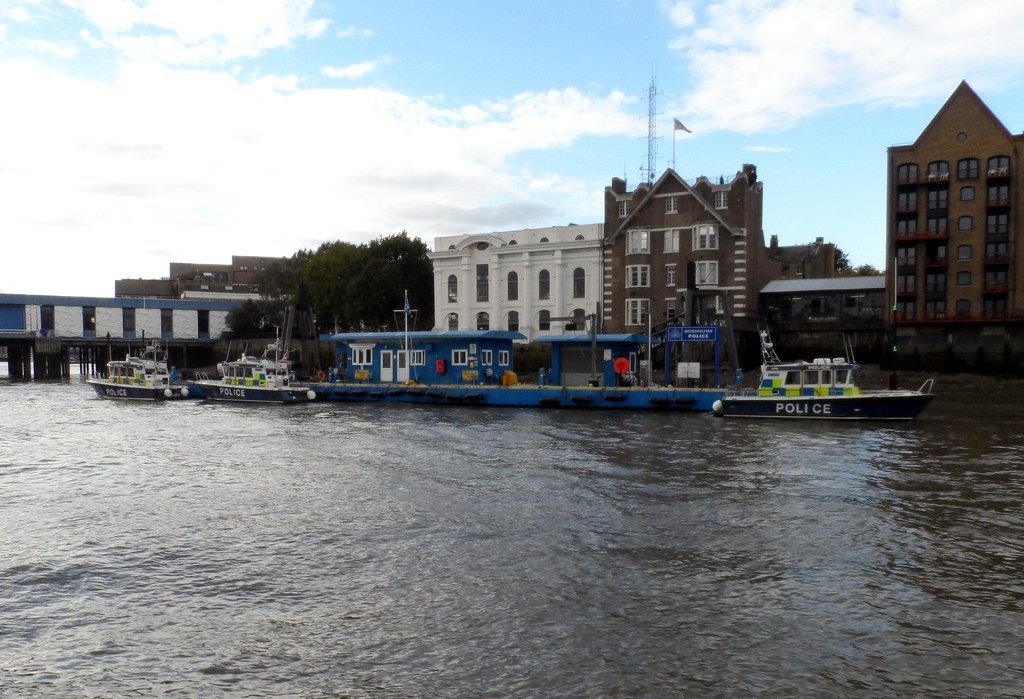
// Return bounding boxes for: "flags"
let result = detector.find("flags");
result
[674,119,691,133]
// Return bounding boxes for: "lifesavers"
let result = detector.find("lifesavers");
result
[318,371,325,380]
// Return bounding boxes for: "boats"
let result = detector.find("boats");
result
[183,341,318,403]
[711,318,940,421]
[84,340,190,402]
